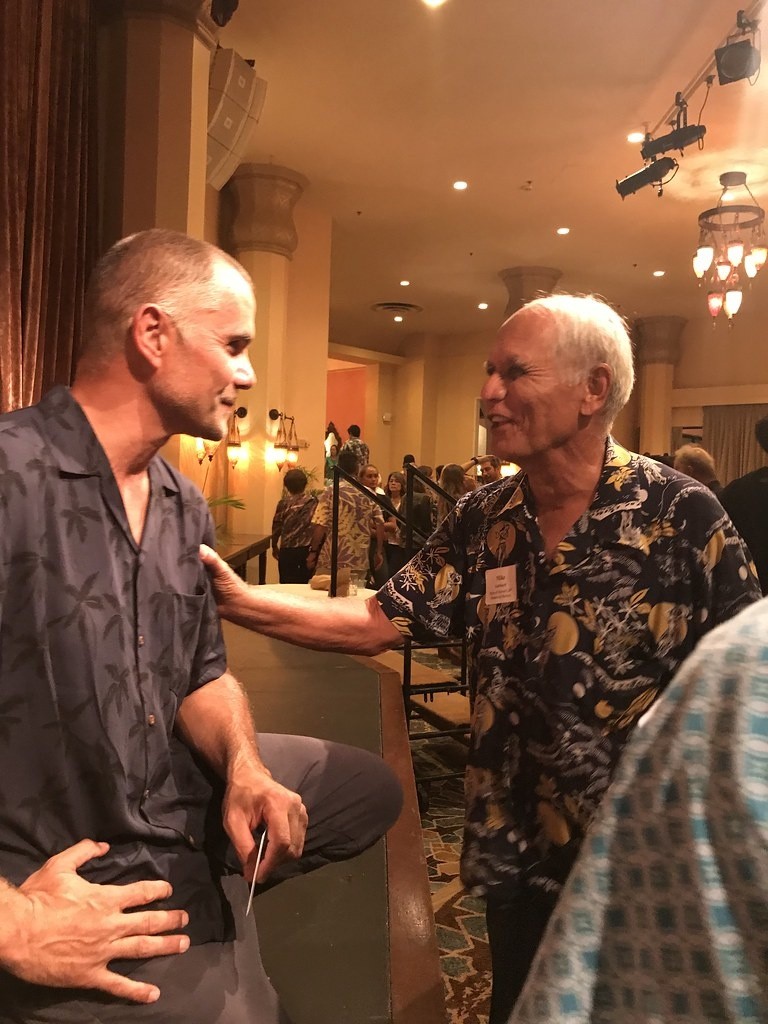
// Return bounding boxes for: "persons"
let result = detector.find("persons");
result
[642,415,767,595]
[200,295,768,1023]
[272,422,503,590]
[0,228,403,1023]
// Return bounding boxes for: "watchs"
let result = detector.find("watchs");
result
[471,457,479,464]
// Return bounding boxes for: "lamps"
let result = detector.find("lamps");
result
[267,409,300,471]
[616,19,761,198]
[691,172,768,327]
[196,408,247,469]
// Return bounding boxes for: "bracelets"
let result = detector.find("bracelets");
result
[375,551,384,555]
[394,527,400,536]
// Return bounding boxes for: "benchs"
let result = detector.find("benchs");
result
[375,640,474,788]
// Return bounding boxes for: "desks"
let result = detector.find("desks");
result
[213,534,272,585]
